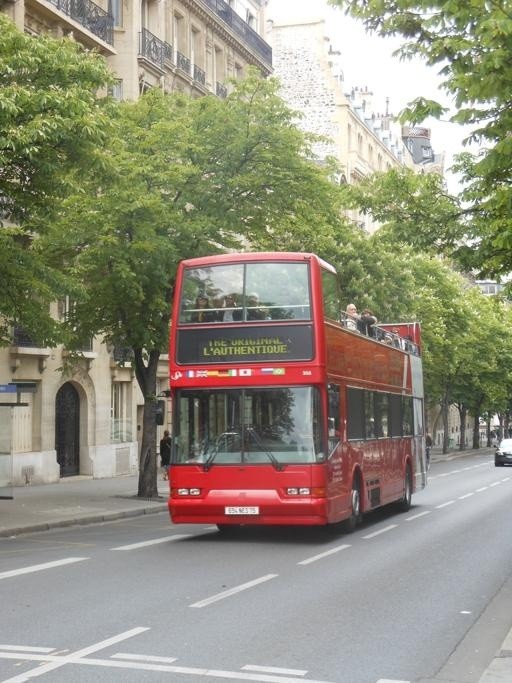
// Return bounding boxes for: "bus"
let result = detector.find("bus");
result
[156,252,428,533]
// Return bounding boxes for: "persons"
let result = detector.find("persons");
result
[425,430,432,471]
[339,302,419,355]
[209,293,226,321]
[190,293,217,322]
[160,429,174,480]
[269,286,302,320]
[217,291,244,322]
[242,292,267,320]
[488,429,497,448]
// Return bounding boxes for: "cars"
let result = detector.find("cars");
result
[494,439,512,466]
